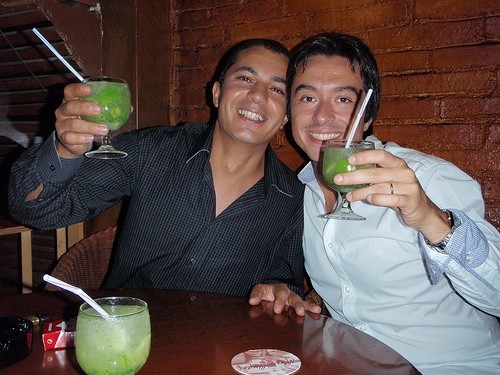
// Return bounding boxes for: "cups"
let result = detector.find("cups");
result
[76,296,151,375]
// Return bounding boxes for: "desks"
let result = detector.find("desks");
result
[0,284,423,375]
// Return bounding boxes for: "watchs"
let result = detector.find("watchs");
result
[425,208,459,250]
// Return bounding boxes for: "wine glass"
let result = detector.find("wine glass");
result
[318,138,376,220]
[77,75,131,159]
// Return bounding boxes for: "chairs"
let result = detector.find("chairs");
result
[0,200,123,295]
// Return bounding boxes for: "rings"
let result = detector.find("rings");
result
[390,182,394,195]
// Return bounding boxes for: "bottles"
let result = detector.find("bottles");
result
[0,312,50,367]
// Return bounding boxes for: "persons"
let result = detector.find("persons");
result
[284,32,500,375]
[6,38,322,317]
